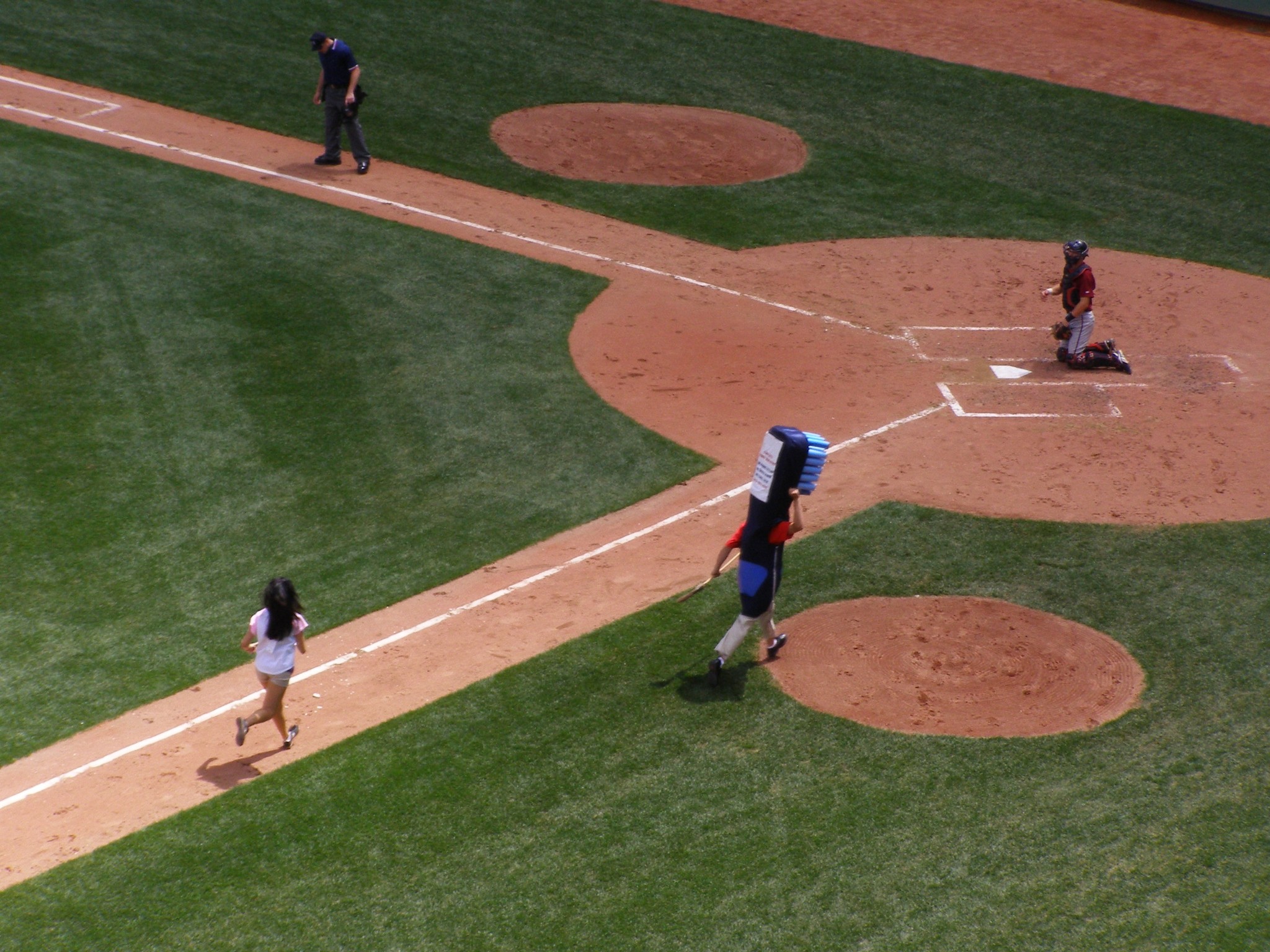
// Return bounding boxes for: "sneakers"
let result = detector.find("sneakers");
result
[1106,338,1117,353]
[1113,350,1132,374]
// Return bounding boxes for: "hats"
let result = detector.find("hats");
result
[309,29,328,51]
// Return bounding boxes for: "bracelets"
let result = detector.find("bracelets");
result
[1065,312,1075,322]
[1047,288,1053,295]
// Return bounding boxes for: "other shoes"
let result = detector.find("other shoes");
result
[767,633,788,658]
[283,724,299,749]
[315,154,341,166]
[358,160,370,174]
[708,658,722,686]
[235,717,249,746]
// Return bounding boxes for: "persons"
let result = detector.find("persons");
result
[707,487,804,688]
[1041,240,1133,375]
[235,576,309,748]
[309,31,371,174]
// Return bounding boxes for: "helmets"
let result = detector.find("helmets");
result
[1063,239,1088,265]
